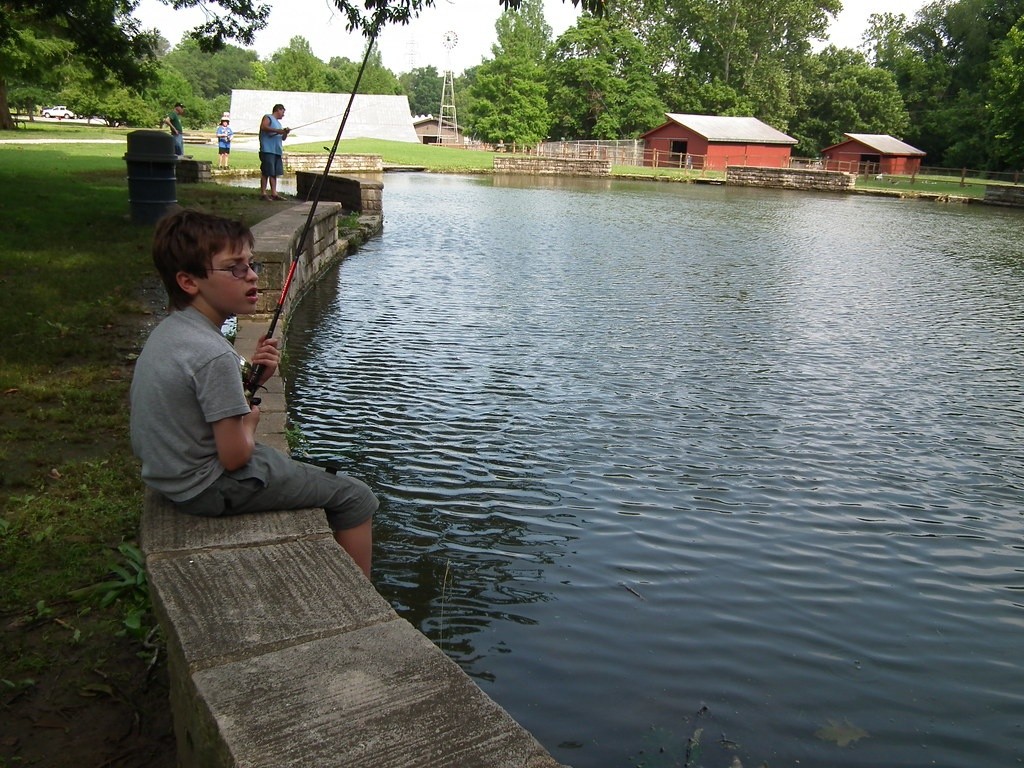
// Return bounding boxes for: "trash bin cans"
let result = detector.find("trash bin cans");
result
[121,129,180,224]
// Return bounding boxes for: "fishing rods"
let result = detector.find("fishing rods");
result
[269,104,373,135]
[245,1,389,402]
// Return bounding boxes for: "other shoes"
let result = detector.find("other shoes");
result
[258,195,273,202]
[270,194,287,201]
[218,165,223,170]
[224,166,230,170]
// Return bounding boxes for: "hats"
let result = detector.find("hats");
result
[174,101,186,109]
[219,117,230,125]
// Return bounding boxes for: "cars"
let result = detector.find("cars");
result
[42,106,74,119]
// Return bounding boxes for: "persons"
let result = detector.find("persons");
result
[165,103,184,156]
[130,210,381,585]
[216,117,233,170]
[258,104,291,201]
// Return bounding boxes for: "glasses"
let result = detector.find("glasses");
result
[202,262,265,279]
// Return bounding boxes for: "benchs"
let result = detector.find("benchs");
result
[10,112,27,130]
